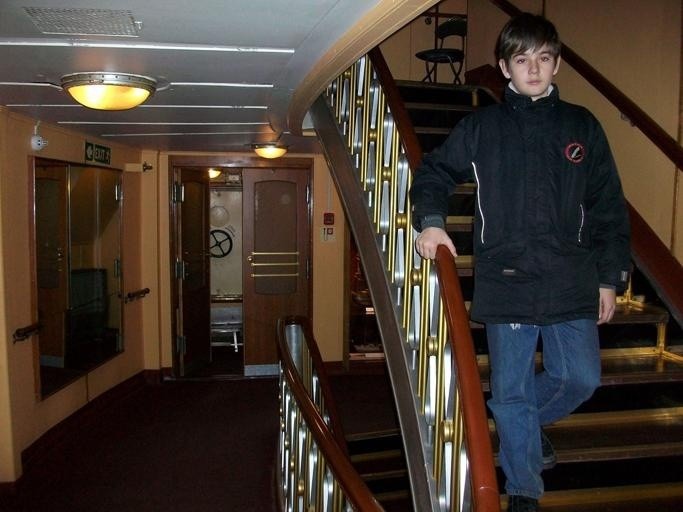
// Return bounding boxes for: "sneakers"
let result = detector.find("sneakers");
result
[507,495,537,512]
[540,432,556,469]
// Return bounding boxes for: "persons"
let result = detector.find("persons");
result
[410,15,632,511]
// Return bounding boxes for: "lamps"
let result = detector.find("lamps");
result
[253,142,289,158]
[60,71,156,111]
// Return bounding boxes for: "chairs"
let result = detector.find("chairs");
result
[414,17,468,85]
